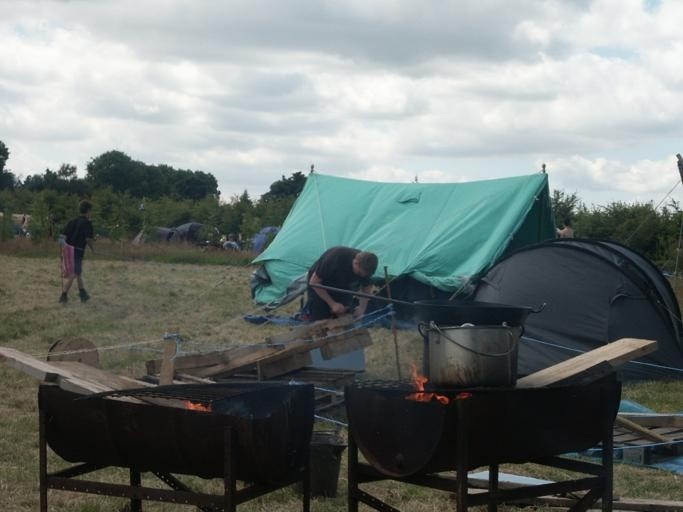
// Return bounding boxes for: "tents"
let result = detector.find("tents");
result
[469,238,681,383]
[244,163,556,306]
[158,222,281,253]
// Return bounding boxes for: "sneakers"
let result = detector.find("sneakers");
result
[58,296,67,303]
[81,295,90,302]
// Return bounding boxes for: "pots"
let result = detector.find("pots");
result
[416,321,526,390]
[308,282,547,327]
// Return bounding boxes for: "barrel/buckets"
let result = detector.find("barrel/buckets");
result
[292,429,348,498]
[417,318,521,390]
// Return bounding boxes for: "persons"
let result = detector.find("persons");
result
[58,201,94,304]
[555,218,573,237]
[305,245,377,322]
